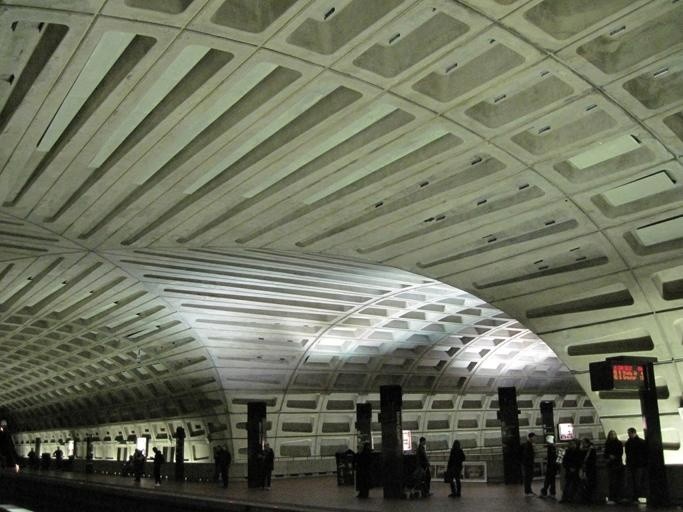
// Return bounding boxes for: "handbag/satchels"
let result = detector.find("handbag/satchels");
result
[443,472,450,483]
[578,469,586,480]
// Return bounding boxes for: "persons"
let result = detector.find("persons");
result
[622,427,645,503]
[53,446,62,468]
[120,446,164,485]
[602,430,624,505]
[255,443,264,488]
[213,444,224,483]
[519,432,536,497]
[578,437,598,503]
[219,445,231,490]
[27,448,35,469]
[262,442,274,491]
[349,433,372,499]
[416,437,434,497]
[537,435,558,500]
[445,440,465,497]
[558,437,582,503]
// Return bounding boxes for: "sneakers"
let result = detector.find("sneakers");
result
[525,492,555,499]
[448,493,461,498]
